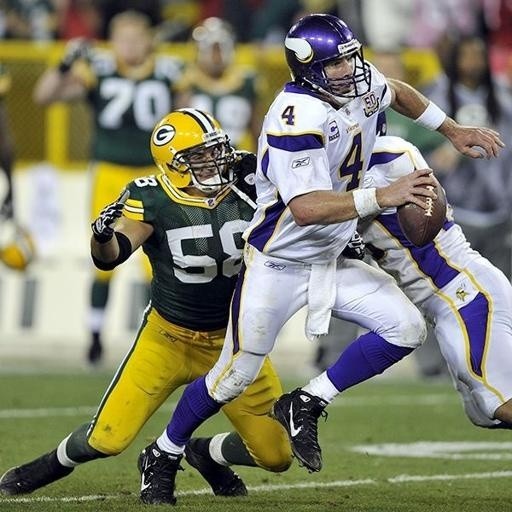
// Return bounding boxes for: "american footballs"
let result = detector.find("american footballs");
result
[395,174,446,247]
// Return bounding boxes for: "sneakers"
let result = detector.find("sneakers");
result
[0,449,74,495]
[274,386,329,474]
[184,438,248,495]
[137,440,183,505]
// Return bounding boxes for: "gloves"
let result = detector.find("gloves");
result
[91,190,131,237]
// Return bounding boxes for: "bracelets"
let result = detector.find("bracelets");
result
[412,99,447,131]
[351,187,387,220]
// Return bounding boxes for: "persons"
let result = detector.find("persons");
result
[356,111,512,430]
[0,105,297,497]
[416,26,512,375]
[371,55,462,175]
[175,12,272,153]
[2,0,511,50]
[135,10,506,506]
[0,69,66,289]
[29,8,241,362]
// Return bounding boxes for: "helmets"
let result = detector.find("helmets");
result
[284,15,363,95]
[150,107,229,188]
